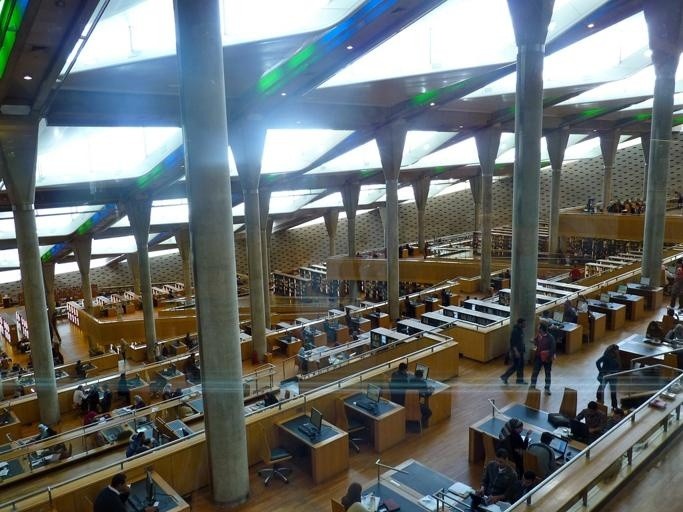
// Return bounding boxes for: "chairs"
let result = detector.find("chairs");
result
[404,388,424,434]
[334,397,367,456]
[1,264,681,392]
[255,421,296,488]
[466,385,624,490]
[1,379,302,490]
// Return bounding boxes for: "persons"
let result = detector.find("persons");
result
[564,300,577,323]
[390,362,408,407]
[528,322,556,395]
[477,448,517,503]
[339,482,376,512]
[72,331,202,456]
[93,473,156,512]
[0,352,26,375]
[646,310,683,343]
[596,343,621,410]
[27,425,67,455]
[569,264,581,282]
[498,419,558,478]
[404,289,449,316]
[499,318,529,385]
[675,191,683,208]
[504,469,538,506]
[573,401,625,434]
[661,257,683,309]
[399,243,414,258]
[407,370,435,429]
[586,197,645,215]
[323,310,356,341]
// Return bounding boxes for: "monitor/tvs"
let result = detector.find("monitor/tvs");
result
[552,276,651,324]
[415,362,429,380]
[146,471,156,501]
[311,407,322,430]
[367,383,379,403]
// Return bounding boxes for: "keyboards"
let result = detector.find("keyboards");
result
[356,401,373,411]
[298,425,315,436]
[128,494,146,512]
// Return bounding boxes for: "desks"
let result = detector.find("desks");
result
[114,469,190,512]
[340,389,408,454]
[340,457,512,512]
[275,410,350,486]
[407,371,452,428]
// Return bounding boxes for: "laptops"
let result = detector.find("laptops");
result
[549,435,568,460]
[469,493,498,506]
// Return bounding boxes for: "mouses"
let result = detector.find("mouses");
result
[374,411,377,415]
[310,436,315,440]
